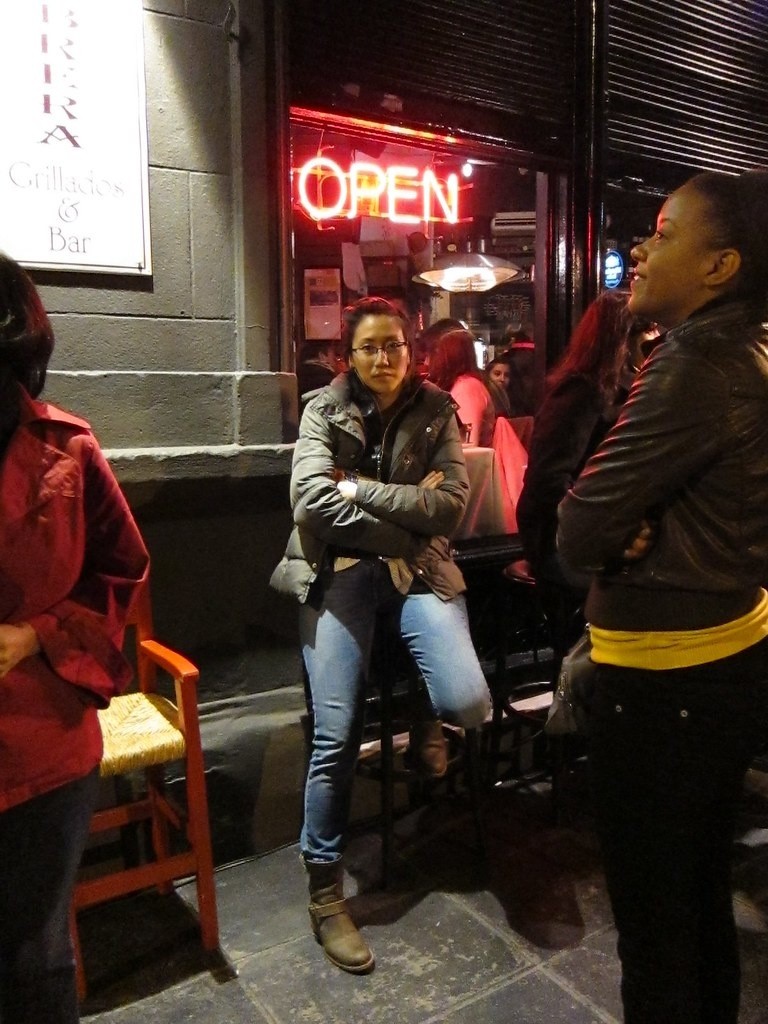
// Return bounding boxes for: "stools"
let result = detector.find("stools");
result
[301,569,587,904]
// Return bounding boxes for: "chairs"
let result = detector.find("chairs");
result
[64,570,221,998]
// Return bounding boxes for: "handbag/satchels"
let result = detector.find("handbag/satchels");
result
[545,629,596,737]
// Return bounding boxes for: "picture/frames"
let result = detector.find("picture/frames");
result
[301,264,344,342]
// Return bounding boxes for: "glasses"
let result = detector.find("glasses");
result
[350,340,409,356]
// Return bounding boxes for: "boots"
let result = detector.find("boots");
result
[408,676,448,777]
[306,858,376,973]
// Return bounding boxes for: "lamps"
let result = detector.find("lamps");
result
[417,234,528,297]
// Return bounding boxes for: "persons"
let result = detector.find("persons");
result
[268,298,493,977]
[0,255,153,1024]
[553,168,768,1024]
[299,291,643,593]
